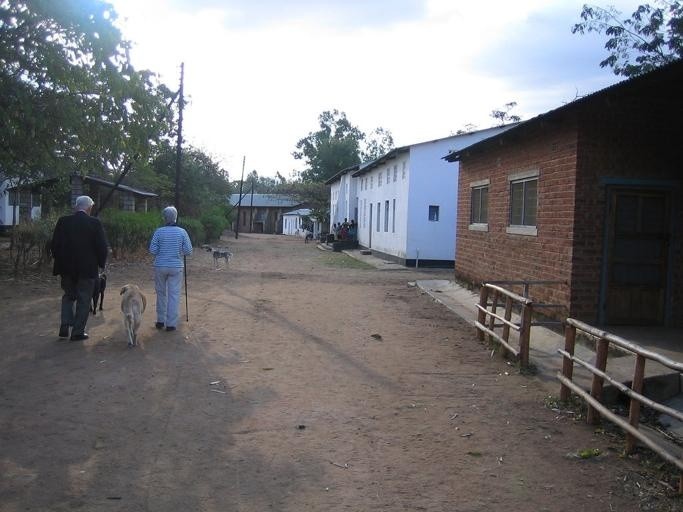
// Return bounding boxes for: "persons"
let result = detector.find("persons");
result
[333,218,357,241]
[51,196,108,341]
[148,206,193,330]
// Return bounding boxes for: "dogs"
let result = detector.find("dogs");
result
[89,272,107,315]
[120,284,146,349]
[201,245,232,266]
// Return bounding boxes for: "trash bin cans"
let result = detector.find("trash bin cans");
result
[320,236,326,243]
[326,234,334,242]
[332,243,342,252]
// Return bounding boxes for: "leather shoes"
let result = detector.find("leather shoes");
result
[155,321,165,328]
[166,326,176,331]
[71,333,88,340]
[58,330,69,336]
